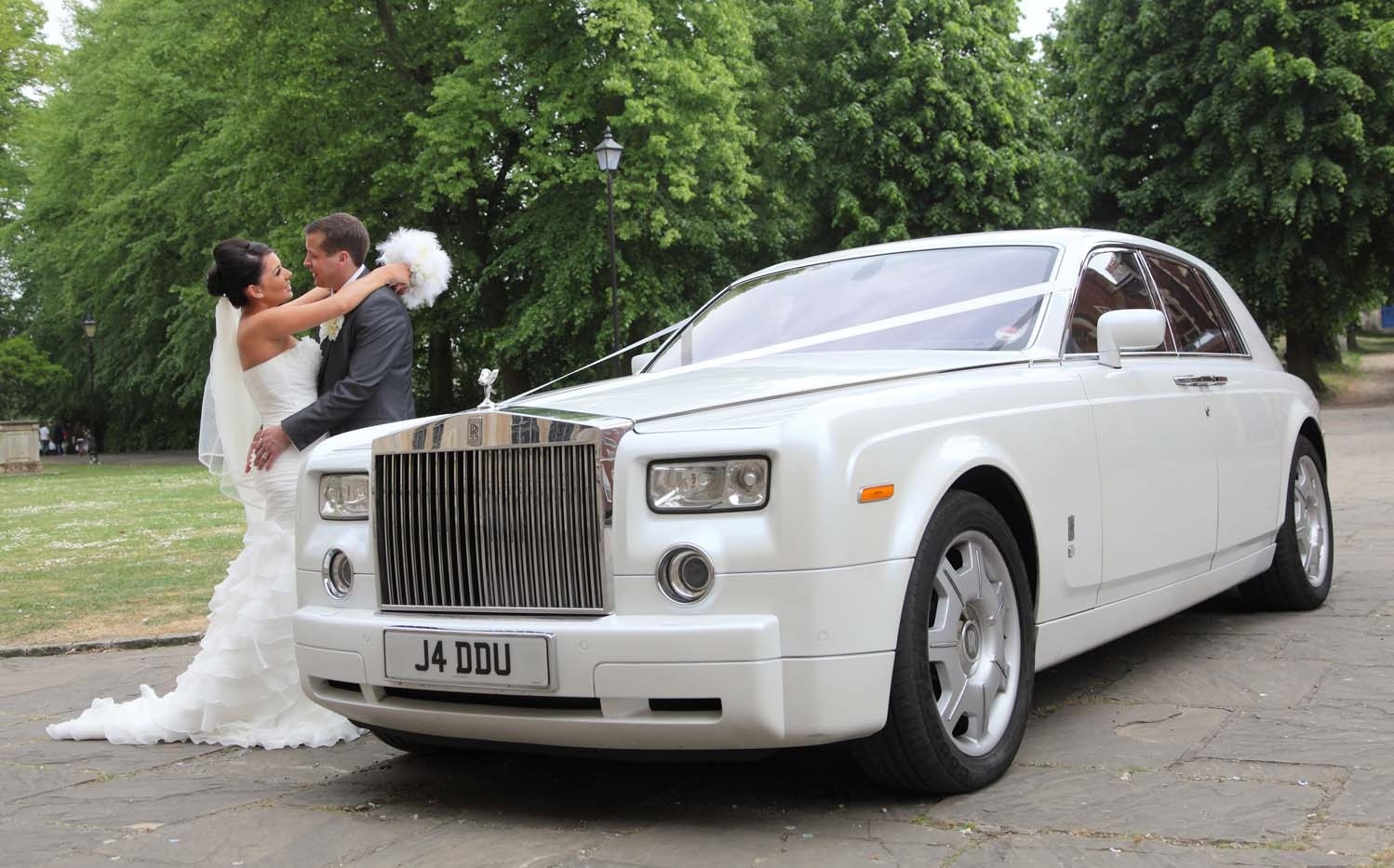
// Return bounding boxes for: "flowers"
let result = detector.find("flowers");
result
[317,314,346,346]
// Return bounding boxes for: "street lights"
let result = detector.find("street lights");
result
[593,126,626,376]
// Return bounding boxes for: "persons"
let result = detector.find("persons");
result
[46,237,412,751]
[244,211,413,476]
[39,423,84,456]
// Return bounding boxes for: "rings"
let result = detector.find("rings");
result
[262,453,268,459]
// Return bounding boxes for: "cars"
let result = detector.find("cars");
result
[295,225,1334,800]
